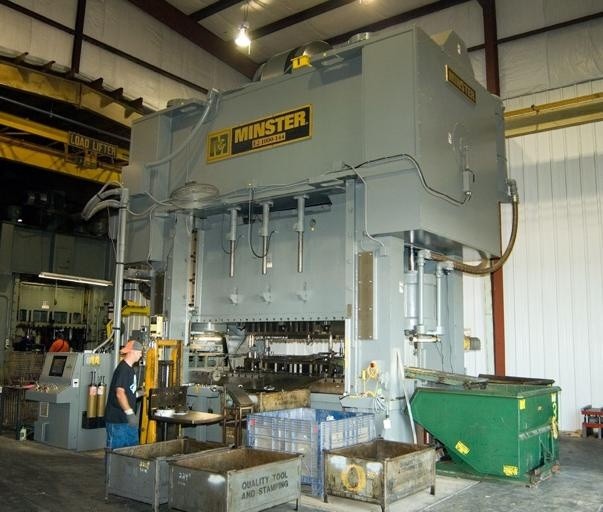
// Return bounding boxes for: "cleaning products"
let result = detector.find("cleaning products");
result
[19,426,27,441]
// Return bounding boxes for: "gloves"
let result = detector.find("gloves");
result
[125,409,138,426]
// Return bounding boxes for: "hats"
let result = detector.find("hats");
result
[121,341,143,354]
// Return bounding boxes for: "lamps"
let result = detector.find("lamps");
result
[230,2,252,50]
[36,209,114,288]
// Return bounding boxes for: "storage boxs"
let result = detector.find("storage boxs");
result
[245,409,379,496]
[321,431,440,511]
[164,440,305,511]
[103,436,231,510]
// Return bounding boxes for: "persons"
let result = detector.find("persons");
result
[103,339,143,477]
[47,333,68,352]
